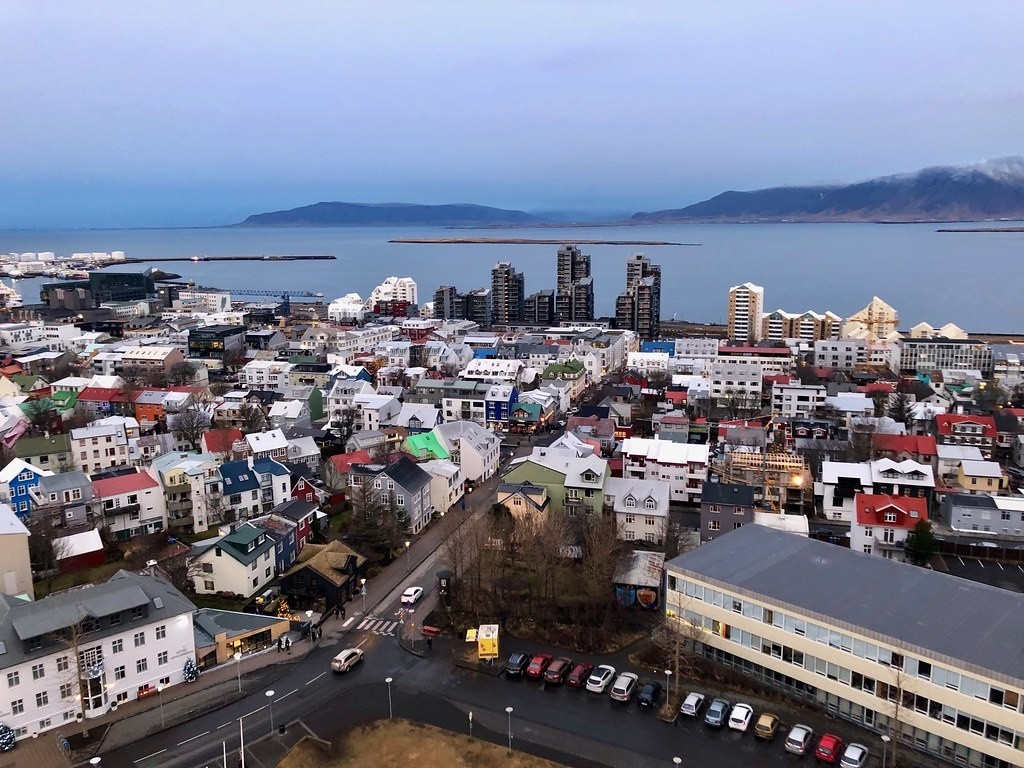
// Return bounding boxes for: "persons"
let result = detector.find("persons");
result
[496,467,500,475]
[335,606,346,620]
[312,625,323,642]
[533,428,555,439]
[461,501,466,511]
[276,637,290,653]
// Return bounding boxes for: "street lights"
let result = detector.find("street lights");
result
[360,578,367,611]
[405,541,411,572]
[156,687,164,728]
[505,707,514,755]
[385,677,393,720]
[665,669,672,711]
[409,609,415,648]
[306,609,313,644]
[265,689,276,737]
[233,651,242,693]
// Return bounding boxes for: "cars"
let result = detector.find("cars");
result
[611,671,639,703]
[543,656,574,685]
[681,692,705,718]
[754,712,781,741]
[526,653,553,679]
[729,703,754,733]
[565,661,594,689]
[585,664,616,695]
[400,586,424,605]
[704,698,731,730]
[637,681,661,711]
[837,743,869,768]
[784,723,815,757]
[331,648,365,673]
[558,366,620,433]
[815,733,843,764]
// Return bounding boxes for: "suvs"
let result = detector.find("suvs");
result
[505,648,532,679]
